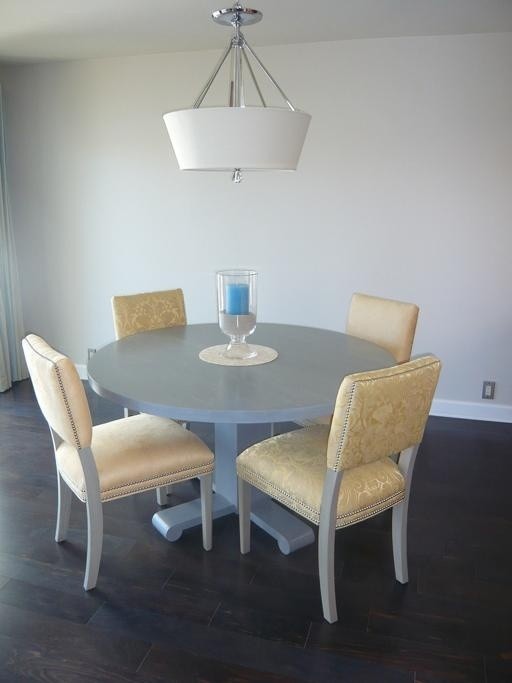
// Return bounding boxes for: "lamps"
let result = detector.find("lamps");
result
[162,0,313,183]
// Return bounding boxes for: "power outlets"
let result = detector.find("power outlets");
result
[482,381,496,400]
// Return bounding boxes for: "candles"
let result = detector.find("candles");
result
[226,283,250,315]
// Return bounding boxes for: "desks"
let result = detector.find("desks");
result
[85,321,398,557]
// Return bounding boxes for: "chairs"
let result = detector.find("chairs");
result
[234,349,444,622]
[20,331,218,597]
[108,287,190,511]
[288,289,420,430]
